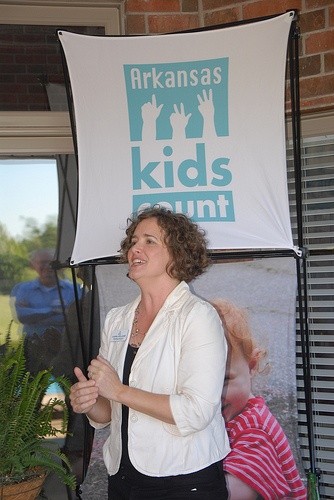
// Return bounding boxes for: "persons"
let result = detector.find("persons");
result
[69,206,231,500]
[204,295,306,500]
[15,248,100,500]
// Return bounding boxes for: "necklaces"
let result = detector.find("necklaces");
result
[131,302,146,354]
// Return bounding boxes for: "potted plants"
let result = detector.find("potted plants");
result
[0,317,76,500]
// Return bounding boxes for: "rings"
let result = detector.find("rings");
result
[87,371,93,379]
